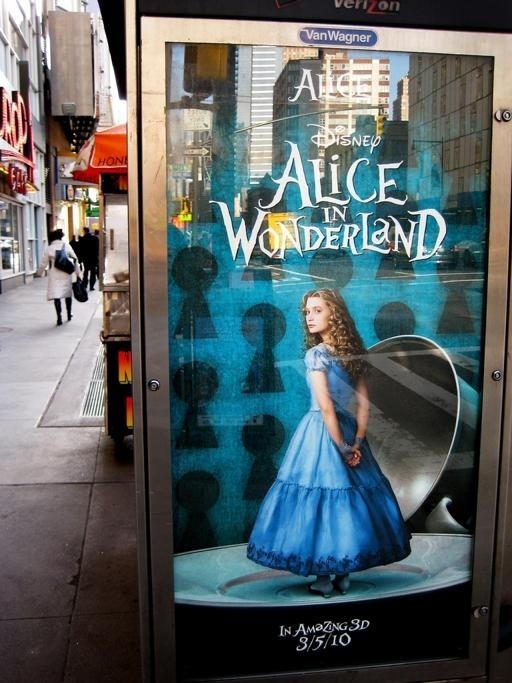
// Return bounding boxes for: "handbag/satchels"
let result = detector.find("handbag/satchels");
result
[71,276,88,302]
[55,242,77,274]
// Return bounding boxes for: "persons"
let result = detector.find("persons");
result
[243,287,411,595]
[34,227,100,326]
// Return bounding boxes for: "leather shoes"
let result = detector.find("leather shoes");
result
[67,311,73,321]
[57,315,63,326]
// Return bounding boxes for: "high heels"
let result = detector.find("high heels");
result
[309,579,334,598]
[330,578,351,595]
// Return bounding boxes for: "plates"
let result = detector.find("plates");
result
[173,532,474,608]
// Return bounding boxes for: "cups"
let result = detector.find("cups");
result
[349,334,480,533]
